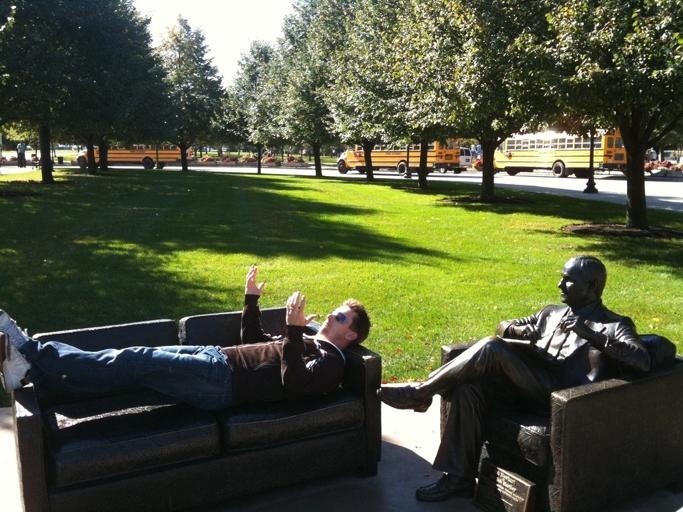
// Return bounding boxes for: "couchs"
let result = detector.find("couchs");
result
[15,310,383,512]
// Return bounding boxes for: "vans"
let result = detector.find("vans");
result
[438,146,473,174]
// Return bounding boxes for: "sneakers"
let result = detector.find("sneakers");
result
[0,331,32,394]
[0,308,30,351]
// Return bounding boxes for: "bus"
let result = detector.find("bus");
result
[475,127,628,178]
[76,143,195,170]
[336,137,460,176]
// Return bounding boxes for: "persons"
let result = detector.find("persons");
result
[17,139,27,167]
[0,263,370,408]
[477,142,482,155]
[470,141,477,163]
[376,256,654,502]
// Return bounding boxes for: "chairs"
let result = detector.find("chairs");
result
[435,332,680,509]
